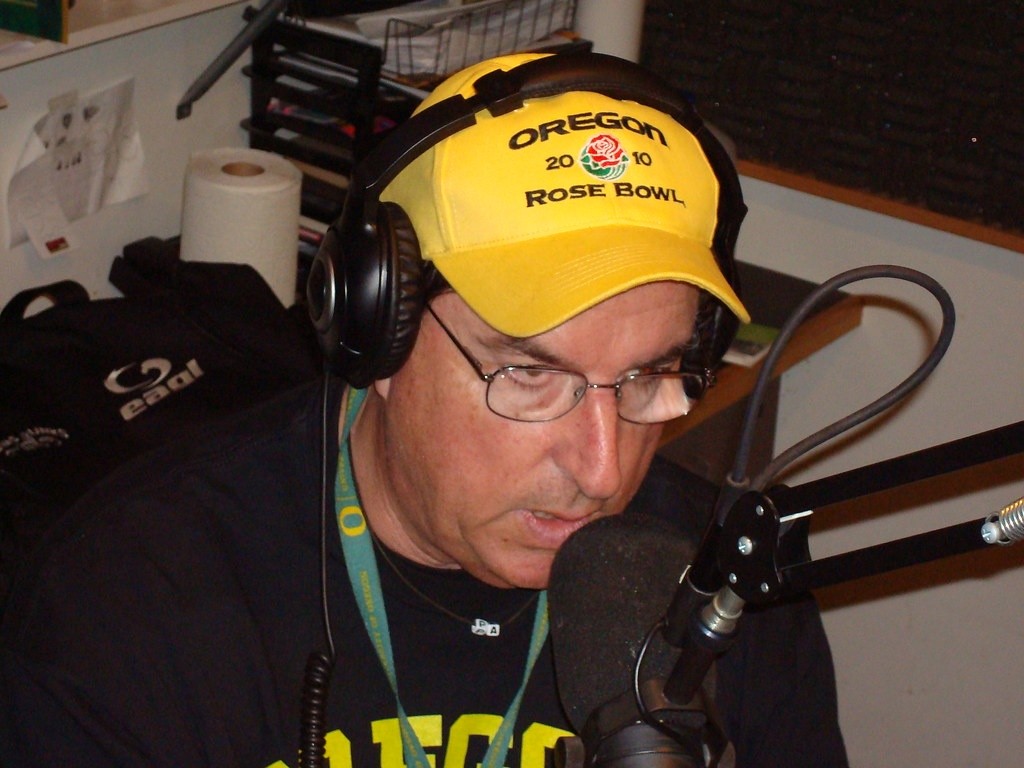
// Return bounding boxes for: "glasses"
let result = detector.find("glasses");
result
[423,298,715,425]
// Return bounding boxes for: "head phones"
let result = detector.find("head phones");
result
[308,52,748,404]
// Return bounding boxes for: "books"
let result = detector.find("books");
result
[720,322,780,368]
[262,1,576,296]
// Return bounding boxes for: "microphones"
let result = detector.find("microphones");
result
[546,512,719,768]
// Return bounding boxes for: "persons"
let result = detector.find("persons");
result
[0,45,853,768]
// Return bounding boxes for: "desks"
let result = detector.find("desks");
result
[652,254,864,492]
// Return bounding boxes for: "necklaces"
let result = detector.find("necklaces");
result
[347,436,542,636]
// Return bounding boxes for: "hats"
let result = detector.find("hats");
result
[379,52,751,337]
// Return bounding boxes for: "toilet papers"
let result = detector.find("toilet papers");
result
[180,146,303,310]
